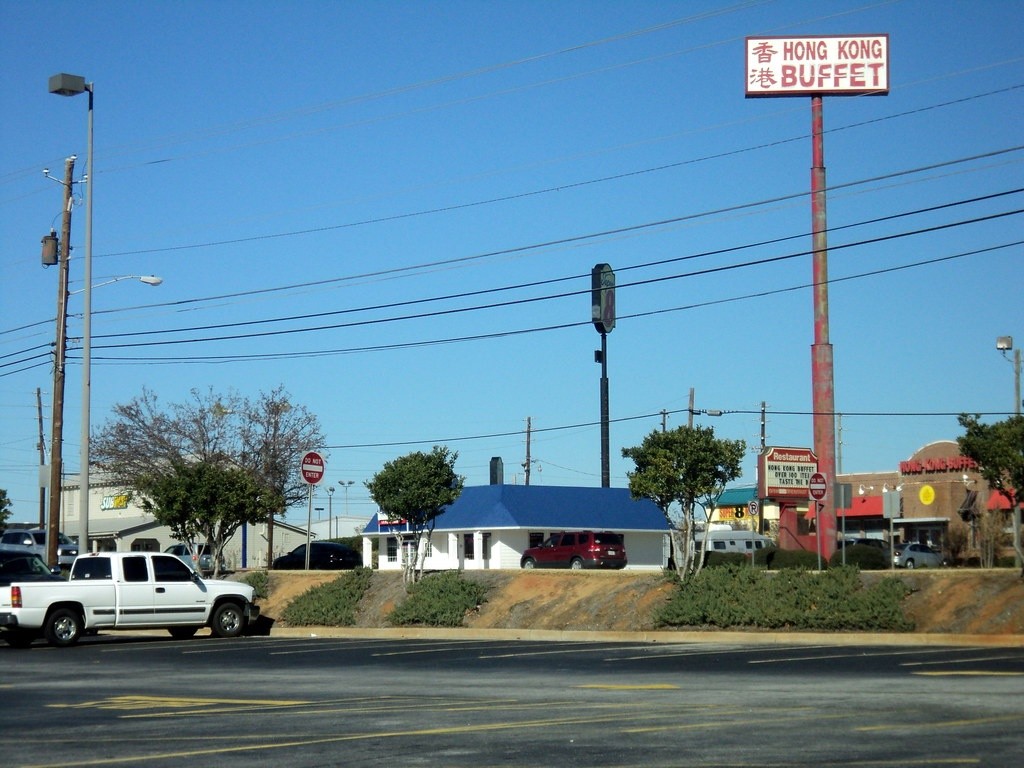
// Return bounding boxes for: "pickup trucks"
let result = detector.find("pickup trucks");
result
[1,551,261,647]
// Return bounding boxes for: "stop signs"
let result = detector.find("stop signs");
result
[808,472,827,501]
[300,449,326,486]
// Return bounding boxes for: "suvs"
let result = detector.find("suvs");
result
[836,537,892,556]
[520,530,629,569]
[164,543,226,575]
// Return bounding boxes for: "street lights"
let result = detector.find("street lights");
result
[47,272,163,574]
[45,70,95,556]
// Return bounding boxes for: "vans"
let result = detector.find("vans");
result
[1,530,78,572]
[691,529,776,555]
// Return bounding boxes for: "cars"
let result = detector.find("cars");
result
[272,541,362,570]
[894,543,948,569]
